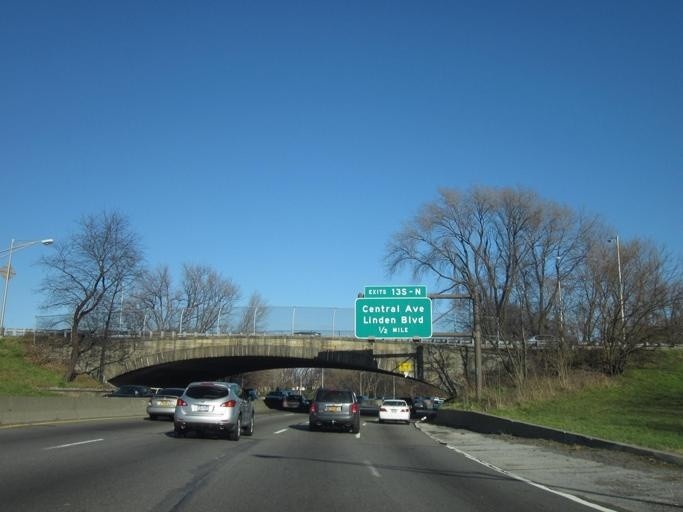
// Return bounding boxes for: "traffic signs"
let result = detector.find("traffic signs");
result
[363,285,428,299]
[353,297,433,340]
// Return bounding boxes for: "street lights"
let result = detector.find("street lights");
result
[0,235,55,334]
[553,255,565,329]
[607,233,628,342]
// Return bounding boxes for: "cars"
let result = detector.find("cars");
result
[169,379,256,441]
[526,332,559,344]
[585,336,619,346]
[293,330,321,336]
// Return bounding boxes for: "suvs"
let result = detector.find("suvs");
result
[308,386,362,434]
